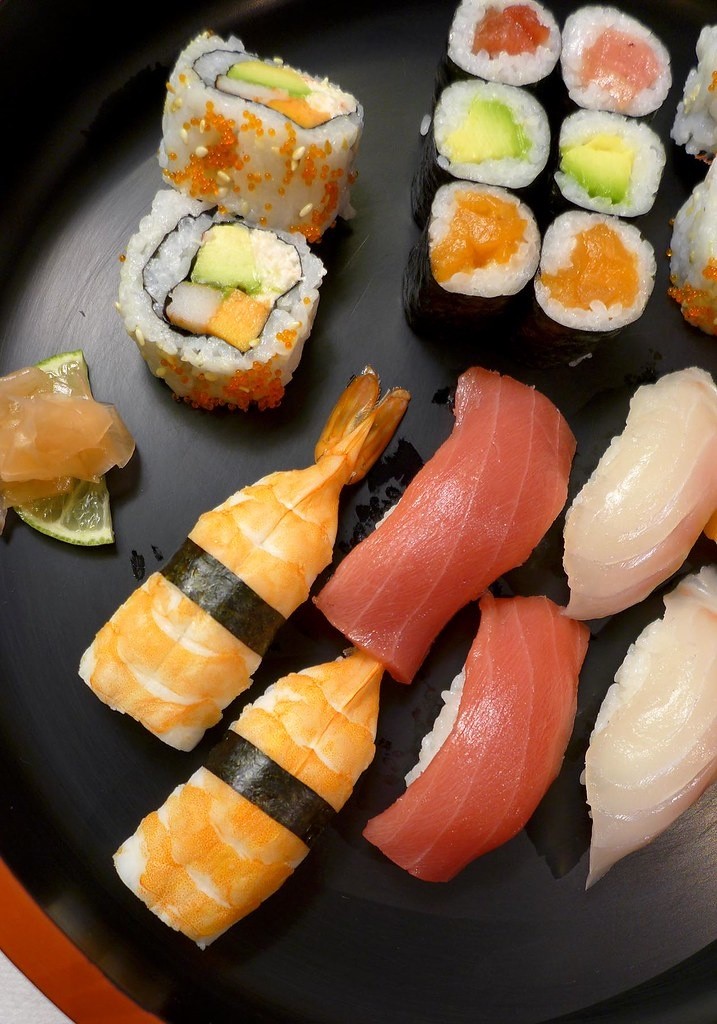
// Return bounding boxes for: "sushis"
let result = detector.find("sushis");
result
[79,0,717,949]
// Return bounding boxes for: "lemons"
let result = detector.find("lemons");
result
[8,351,113,546]
